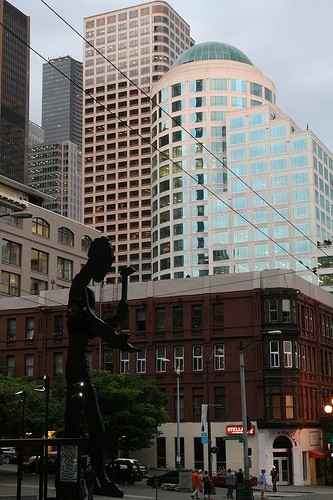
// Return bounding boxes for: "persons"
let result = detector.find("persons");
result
[225,467,243,498]
[258,469,268,498]
[63,236,143,500]
[191,469,212,500]
[270,466,278,493]
[84,463,101,500]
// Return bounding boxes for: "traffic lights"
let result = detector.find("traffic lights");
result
[322,397,333,417]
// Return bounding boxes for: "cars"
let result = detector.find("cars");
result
[0,445,146,485]
[210,469,258,489]
[146,467,193,489]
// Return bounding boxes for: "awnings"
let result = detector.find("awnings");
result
[309,450,327,458]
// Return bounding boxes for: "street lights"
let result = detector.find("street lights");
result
[235,329,282,485]
[14,390,26,500]
[32,374,50,500]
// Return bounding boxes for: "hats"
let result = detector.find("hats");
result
[198,469,202,473]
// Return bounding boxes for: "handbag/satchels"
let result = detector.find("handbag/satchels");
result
[233,475,237,483]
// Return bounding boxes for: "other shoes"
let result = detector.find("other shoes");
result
[191,495,194,499]
[196,497,200,499]
[261,497,266,499]
